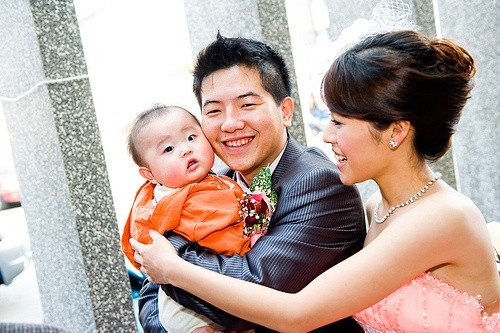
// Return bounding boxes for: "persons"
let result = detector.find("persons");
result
[129,0,500,333]
[139,31,366,333]
[121,104,255,333]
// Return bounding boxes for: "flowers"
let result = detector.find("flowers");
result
[236,163,277,239]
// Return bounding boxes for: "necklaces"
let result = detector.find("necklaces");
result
[374,172,443,223]
